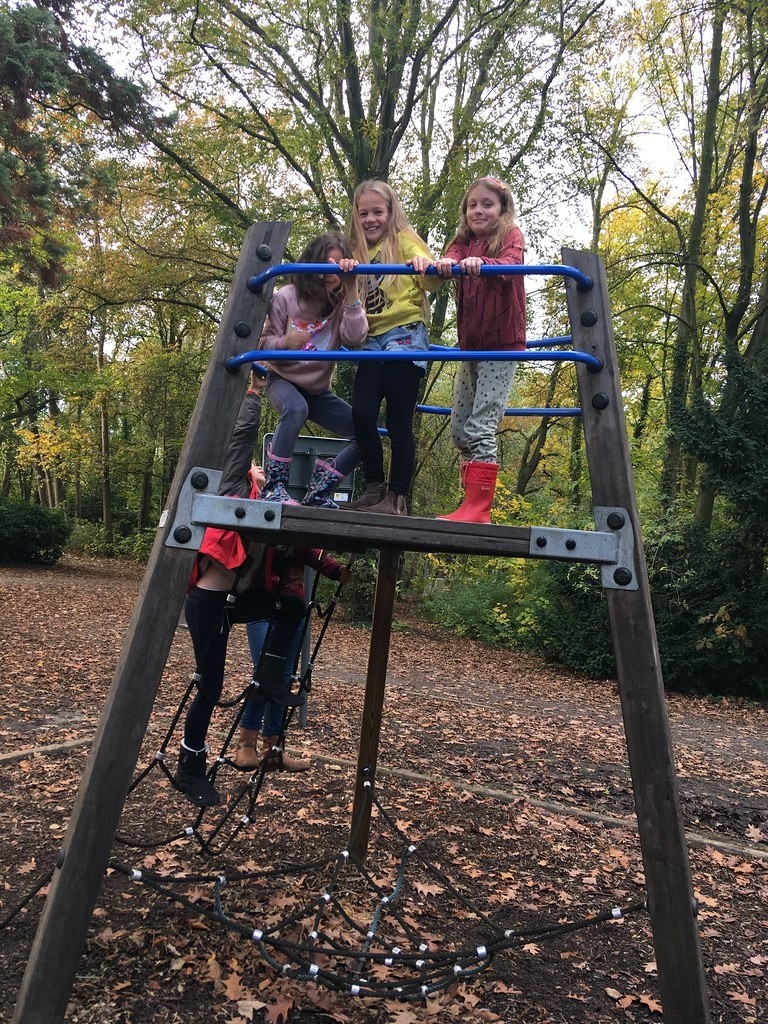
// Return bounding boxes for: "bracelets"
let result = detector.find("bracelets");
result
[277,334,284,349]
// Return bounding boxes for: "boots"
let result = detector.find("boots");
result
[260,441,302,506]
[339,482,408,516]
[435,460,499,524]
[170,738,220,808]
[262,732,311,772]
[299,457,340,509]
[234,726,260,770]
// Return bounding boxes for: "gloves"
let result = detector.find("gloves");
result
[337,562,351,585]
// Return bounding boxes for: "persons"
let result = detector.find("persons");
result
[172,364,350,806]
[434,178,526,525]
[348,181,443,515]
[258,231,369,509]
